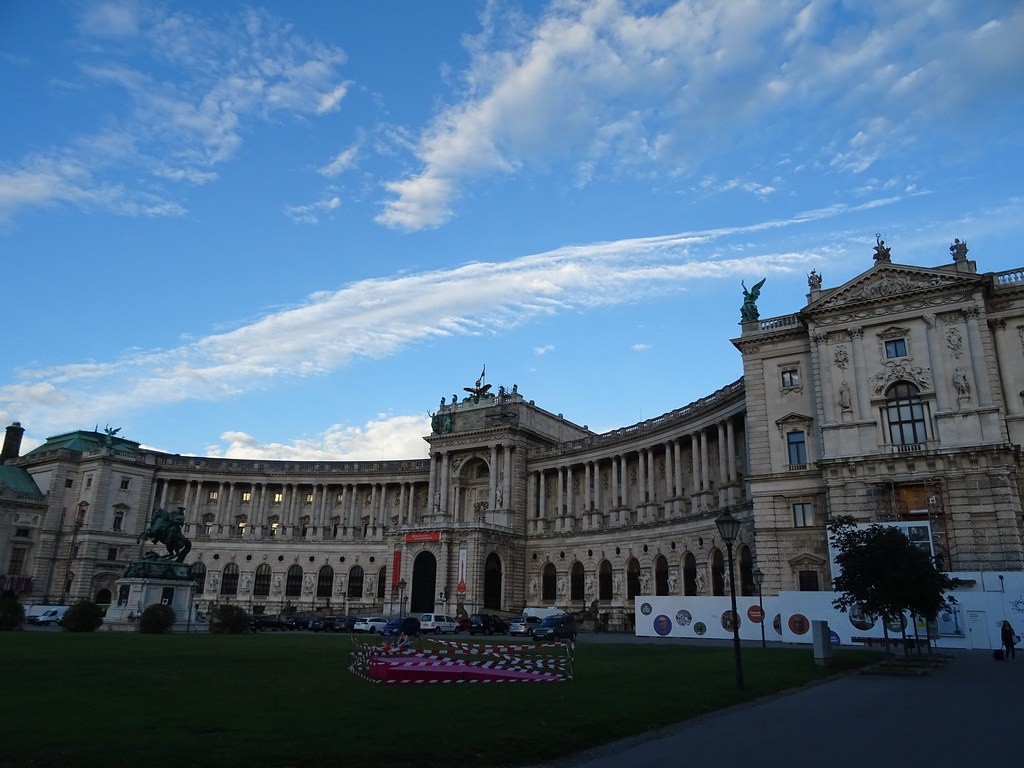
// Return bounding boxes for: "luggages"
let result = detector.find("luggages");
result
[993,644,1005,661]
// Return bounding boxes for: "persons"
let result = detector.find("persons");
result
[305,577,312,591]
[1002,621,1016,663]
[383,631,396,645]
[106,427,112,445]
[586,576,593,591]
[953,367,968,396]
[366,577,373,592]
[240,577,249,591]
[397,632,409,646]
[428,411,439,433]
[556,577,563,593]
[434,489,440,506]
[877,237,886,261]
[162,507,186,540]
[950,238,965,255]
[840,381,850,410]
[496,484,502,502]
[273,576,281,593]
[209,575,216,588]
[739,280,751,318]
[528,577,535,593]
[612,567,730,592]
[336,576,343,591]
[443,414,452,431]
[809,270,820,285]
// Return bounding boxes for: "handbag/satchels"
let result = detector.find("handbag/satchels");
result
[1011,629,1019,645]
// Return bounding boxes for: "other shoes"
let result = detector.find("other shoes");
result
[1013,659,1015,663]
[1006,651,1009,658]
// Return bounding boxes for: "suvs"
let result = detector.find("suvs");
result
[531,612,578,644]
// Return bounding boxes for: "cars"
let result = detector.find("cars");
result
[246,613,359,633]
[353,616,387,634]
[26,606,66,626]
[508,615,543,637]
[379,616,421,637]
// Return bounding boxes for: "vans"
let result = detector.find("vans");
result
[419,613,461,635]
[467,613,509,636]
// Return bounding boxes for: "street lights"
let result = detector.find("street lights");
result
[714,505,746,691]
[184,583,199,632]
[754,568,767,648]
[398,579,407,646]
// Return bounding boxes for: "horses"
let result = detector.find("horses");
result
[136,508,192,562]
